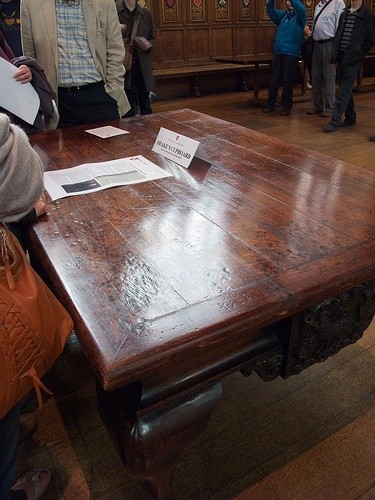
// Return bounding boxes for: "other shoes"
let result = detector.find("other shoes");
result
[320,111,331,116]
[368,136,375,141]
[263,108,274,113]
[281,111,289,115]
[322,122,338,132]
[339,119,356,126]
[307,112,320,114]
[19,414,36,441]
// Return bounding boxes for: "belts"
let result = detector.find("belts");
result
[314,38,334,43]
[58,83,92,92]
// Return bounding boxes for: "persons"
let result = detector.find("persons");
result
[322,0,375,132]
[296,25,312,89]
[0,113,51,500]
[116,0,154,119]
[263,0,306,116]
[307,1,346,117]
[0,31,56,254]
[21,0,131,130]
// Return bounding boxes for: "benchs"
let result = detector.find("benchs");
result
[148,58,272,98]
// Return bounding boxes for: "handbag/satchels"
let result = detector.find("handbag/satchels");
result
[0,221,74,420]
[301,36,314,64]
[123,44,134,71]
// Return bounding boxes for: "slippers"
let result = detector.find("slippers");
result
[11,469,51,500]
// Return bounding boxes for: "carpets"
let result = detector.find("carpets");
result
[47,321,375,499]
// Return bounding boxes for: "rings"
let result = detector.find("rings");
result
[26,74,29,79]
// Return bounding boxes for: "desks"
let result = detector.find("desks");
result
[25,106,375,500]
[218,53,313,102]
